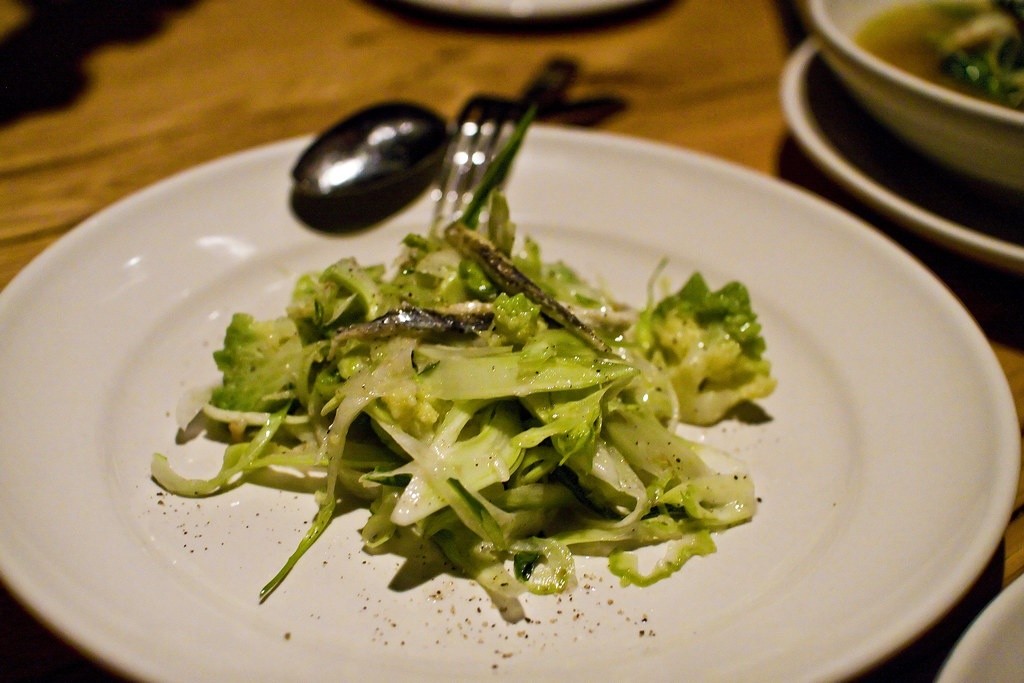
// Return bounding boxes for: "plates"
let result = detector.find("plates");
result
[779,35,1024,272]
[935,573,1024,683]
[0,125,1020,683]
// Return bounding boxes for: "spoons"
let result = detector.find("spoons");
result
[289,96,447,198]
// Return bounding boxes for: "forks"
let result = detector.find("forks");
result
[441,54,578,201]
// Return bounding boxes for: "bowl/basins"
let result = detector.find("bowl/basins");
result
[801,0,1024,194]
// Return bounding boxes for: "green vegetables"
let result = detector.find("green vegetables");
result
[146,107,778,608]
